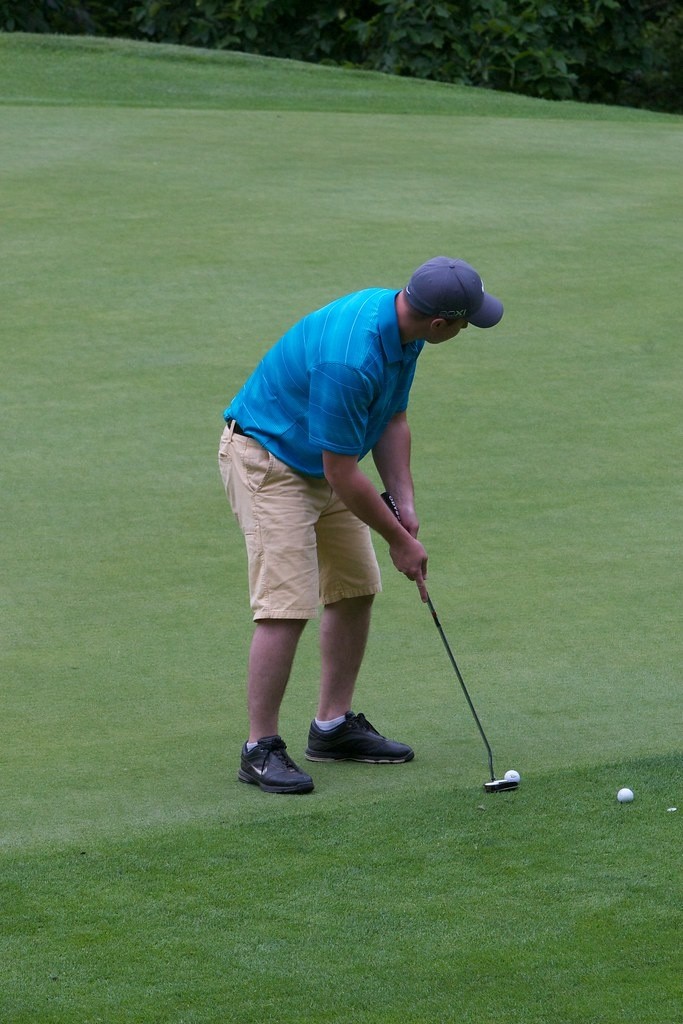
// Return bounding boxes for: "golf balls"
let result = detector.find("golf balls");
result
[616,788,634,804]
[504,770,521,783]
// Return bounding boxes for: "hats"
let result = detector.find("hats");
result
[405,254,505,329]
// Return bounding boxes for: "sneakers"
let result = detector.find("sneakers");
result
[303,710,414,764]
[237,734,314,795]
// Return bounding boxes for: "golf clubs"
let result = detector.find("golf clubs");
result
[380,490,519,794]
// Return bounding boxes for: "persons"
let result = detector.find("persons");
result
[218,255,504,795]
